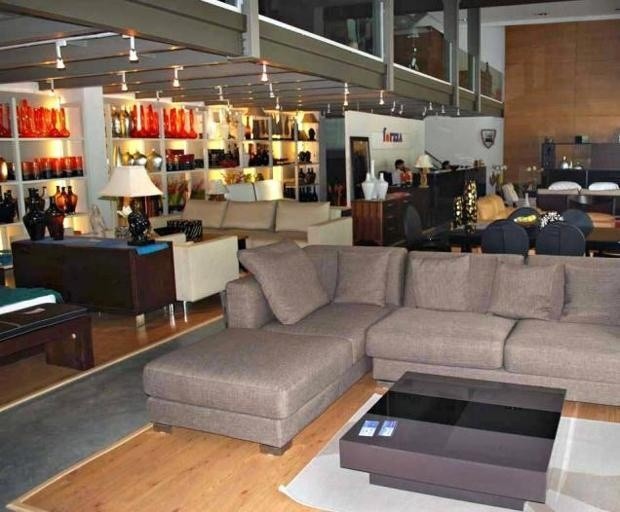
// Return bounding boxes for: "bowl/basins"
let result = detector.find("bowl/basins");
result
[449,165,459,170]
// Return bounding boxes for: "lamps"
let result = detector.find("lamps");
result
[415,153,433,188]
[98,165,164,237]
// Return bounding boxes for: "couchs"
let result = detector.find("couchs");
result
[361,249,620,406]
[139,235,407,458]
[180,198,356,256]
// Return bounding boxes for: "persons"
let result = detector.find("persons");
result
[391,159,405,185]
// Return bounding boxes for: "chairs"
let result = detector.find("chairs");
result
[475,180,620,224]
[535,221,585,255]
[150,232,241,326]
[402,201,450,253]
[476,217,530,255]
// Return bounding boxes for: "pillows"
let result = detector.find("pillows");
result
[561,262,620,320]
[488,258,569,323]
[331,248,391,309]
[234,238,330,324]
[185,198,229,229]
[406,253,475,315]
[223,202,272,232]
[272,198,333,233]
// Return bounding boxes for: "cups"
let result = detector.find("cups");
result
[9,154,84,178]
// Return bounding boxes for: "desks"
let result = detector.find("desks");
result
[444,220,620,253]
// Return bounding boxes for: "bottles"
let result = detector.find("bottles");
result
[111,103,130,136]
[299,167,319,201]
[0,185,78,241]
[249,143,269,166]
[244,116,252,139]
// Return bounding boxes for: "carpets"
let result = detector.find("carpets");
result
[273,390,619,512]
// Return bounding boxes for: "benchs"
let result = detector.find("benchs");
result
[0,302,95,373]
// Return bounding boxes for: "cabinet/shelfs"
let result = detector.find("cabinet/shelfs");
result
[0,91,93,225]
[101,92,164,218]
[350,193,414,249]
[539,139,619,187]
[298,122,324,201]
[9,235,175,331]
[271,118,298,202]
[243,115,271,185]
[208,110,242,193]
[166,99,207,213]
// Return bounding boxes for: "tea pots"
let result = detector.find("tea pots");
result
[558,156,573,169]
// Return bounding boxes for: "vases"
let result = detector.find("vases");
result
[370,160,378,199]
[132,151,147,166]
[362,173,374,200]
[0,98,71,139]
[1,183,79,224]
[120,152,135,166]
[253,150,270,166]
[128,102,200,139]
[44,196,66,241]
[376,172,390,200]
[299,167,319,201]
[146,149,163,171]
[23,200,47,241]
